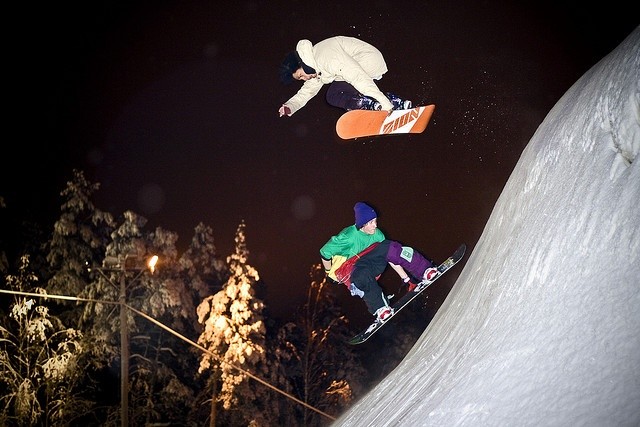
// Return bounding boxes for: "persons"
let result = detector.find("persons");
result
[278,36,413,119]
[319,202,438,321]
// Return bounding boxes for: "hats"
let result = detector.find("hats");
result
[354,202,377,229]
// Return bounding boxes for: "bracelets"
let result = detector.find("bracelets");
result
[325,269,331,273]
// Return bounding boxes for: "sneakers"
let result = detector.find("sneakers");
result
[353,93,382,111]
[389,94,412,109]
[377,306,392,320]
[423,268,438,280]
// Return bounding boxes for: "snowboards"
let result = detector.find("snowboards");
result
[336,104,435,140]
[345,242,466,346]
